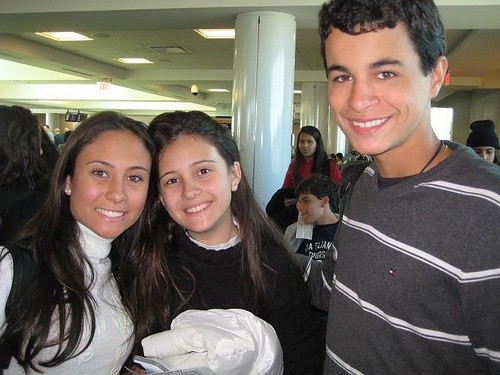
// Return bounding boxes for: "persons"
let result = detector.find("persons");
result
[0,110,158,375]
[109,111,326,375]
[286,0,500,375]
[0,105,500,261]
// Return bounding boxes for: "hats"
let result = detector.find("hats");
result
[465,120,500,150]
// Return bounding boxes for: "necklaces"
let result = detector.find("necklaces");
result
[418,140,445,173]
